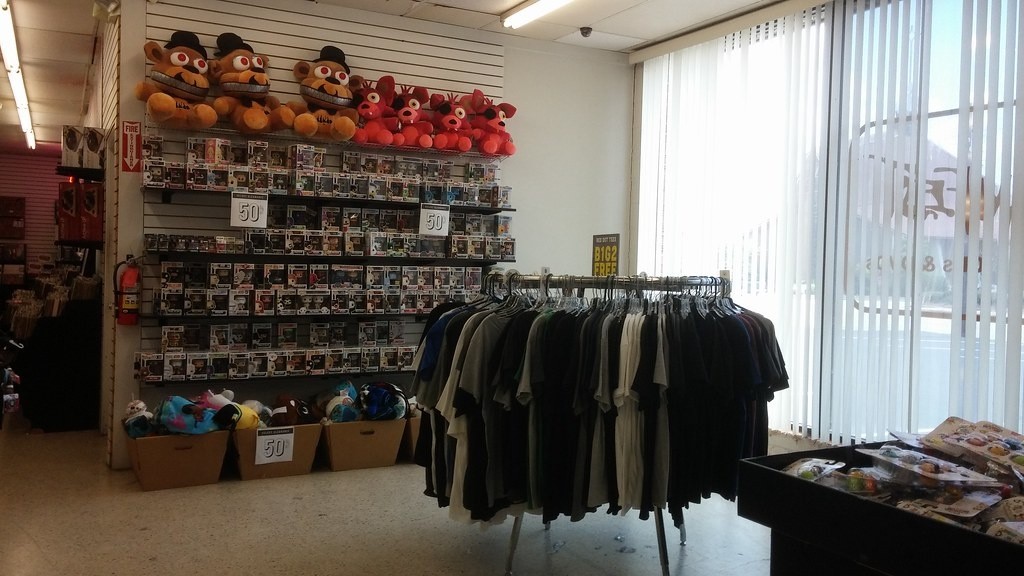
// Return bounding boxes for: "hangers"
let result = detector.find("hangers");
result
[462,273,744,322]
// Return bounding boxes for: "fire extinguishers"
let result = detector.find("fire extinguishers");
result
[112,252,153,326]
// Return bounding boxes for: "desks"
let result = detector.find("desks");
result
[738,439,1024,576]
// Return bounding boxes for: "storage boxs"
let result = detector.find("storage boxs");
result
[163,353,186,381]
[83,127,105,169]
[266,350,287,377]
[141,354,162,381]
[186,352,209,380]
[232,423,324,481]
[81,183,103,240]
[228,352,250,380]
[208,352,228,380]
[60,124,84,168]
[0,196,26,217]
[249,352,269,378]
[2,264,26,285]
[58,181,81,239]
[323,418,407,472]
[133,352,158,380]
[140,134,515,354]
[3,243,26,264]
[402,417,420,459]
[125,430,229,492]
[306,354,419,375]
[287,349,306,376]
[0,217,25,240]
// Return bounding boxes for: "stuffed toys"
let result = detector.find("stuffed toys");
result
[427,93,476,153]
[134,31,217,130]
[383,83,436,147]
[206,33,297,133]
[345,73,400,144]
[473,90,517,155]
[124,380,409,436]
[285,45,362,141]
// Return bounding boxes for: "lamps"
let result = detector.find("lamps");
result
[500,0,567,30]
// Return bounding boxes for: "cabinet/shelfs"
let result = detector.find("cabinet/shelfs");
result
[143,119,516,388]
[53,166,104,251]
